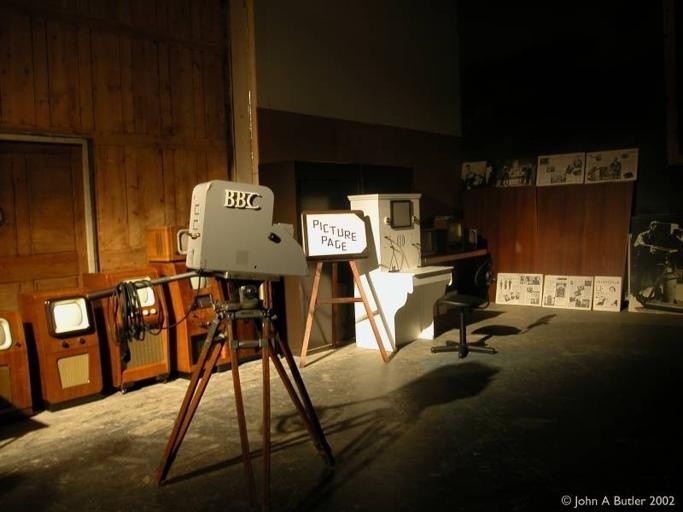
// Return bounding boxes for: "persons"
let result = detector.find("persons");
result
[633,220,683,308]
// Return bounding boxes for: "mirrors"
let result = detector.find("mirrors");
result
[389,199,412,229]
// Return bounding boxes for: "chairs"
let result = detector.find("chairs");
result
[431,252,496,359]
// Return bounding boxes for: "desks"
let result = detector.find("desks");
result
[347,190,454,352]
[421,248,489,266]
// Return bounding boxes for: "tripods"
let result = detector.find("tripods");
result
[154,284,337,512]
